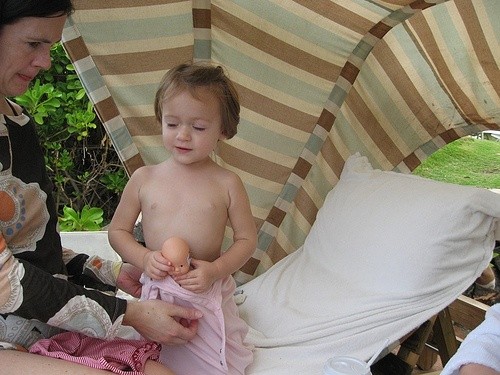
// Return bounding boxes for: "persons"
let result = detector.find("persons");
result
[0,0,203,375]
[439,303,500,374]
[108,62,258,374]
[161,238,194,279]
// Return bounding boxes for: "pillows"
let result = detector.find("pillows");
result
[237,152,500,375]
